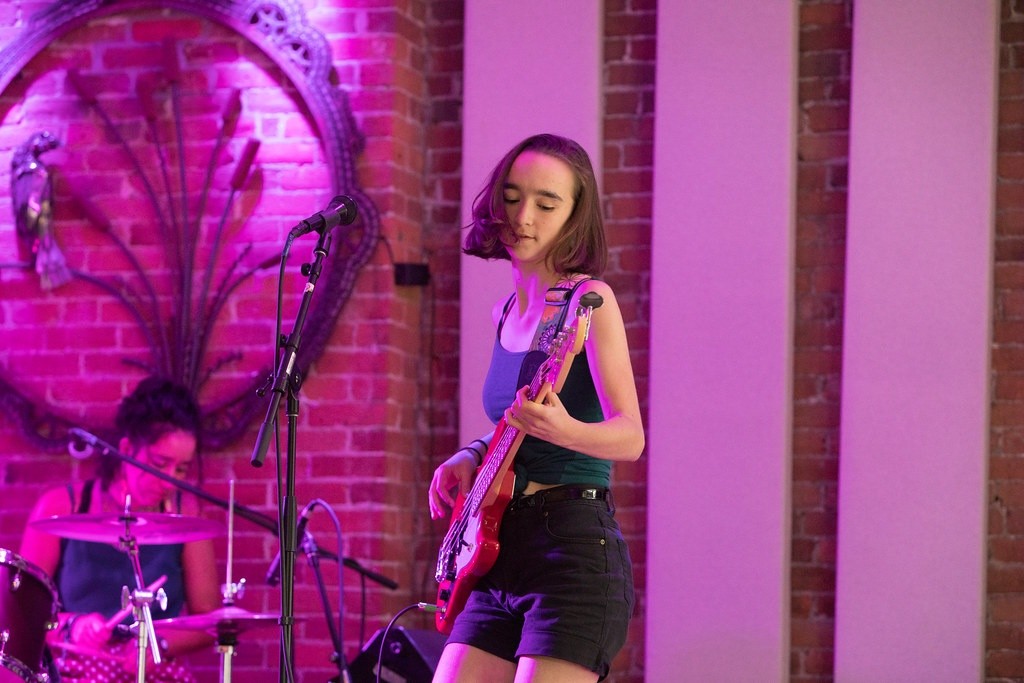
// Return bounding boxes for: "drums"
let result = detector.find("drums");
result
[0,544,61,683]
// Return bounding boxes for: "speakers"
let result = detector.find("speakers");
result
[330,624,448,683]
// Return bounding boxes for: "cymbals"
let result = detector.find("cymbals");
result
[129,594,310,642]
[32,494,220,546]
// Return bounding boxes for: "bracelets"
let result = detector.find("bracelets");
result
[450,447,483,467]
[469,439,488,453]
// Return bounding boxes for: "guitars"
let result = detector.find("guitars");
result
[434,289,608,634]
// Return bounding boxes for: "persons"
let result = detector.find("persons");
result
[23,376,222,683]
[428,134,645,683]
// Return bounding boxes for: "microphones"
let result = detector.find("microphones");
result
[290,194,358,240]
[268,502,315,587]
[68,430,92,460]
